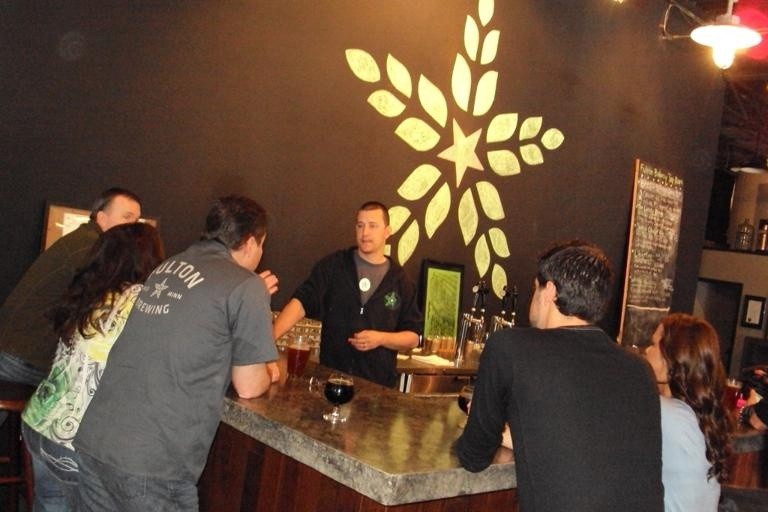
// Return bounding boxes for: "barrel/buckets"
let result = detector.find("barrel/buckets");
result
[735,217,755,252]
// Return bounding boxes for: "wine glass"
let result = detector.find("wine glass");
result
[456,386,474,429]
[321,372,355,423]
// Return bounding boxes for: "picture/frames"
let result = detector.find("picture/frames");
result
[39,200,163,257]
[740,294,766,329]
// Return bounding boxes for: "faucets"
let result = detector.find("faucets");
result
[489,283,519,335]
[455,279,489,369]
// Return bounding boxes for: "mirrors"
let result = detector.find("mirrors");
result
[419,259,465,358]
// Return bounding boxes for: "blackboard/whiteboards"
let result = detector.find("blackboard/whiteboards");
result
[617,158,685,356]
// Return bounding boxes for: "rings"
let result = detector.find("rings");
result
[362,342,367,348]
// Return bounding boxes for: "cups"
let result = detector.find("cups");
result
[421,334,456,357]
[723,378,743,411]
[286,334,314,377]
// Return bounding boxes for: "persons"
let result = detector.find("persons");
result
[449,240,665,512]
[0,188,141,385]
[644,311,736,512]
[72,194,280,512]
[743,396,767,439]
[19,223,165,510]
[272,200,423,388]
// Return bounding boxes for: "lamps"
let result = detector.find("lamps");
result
[690,0,762,70]
